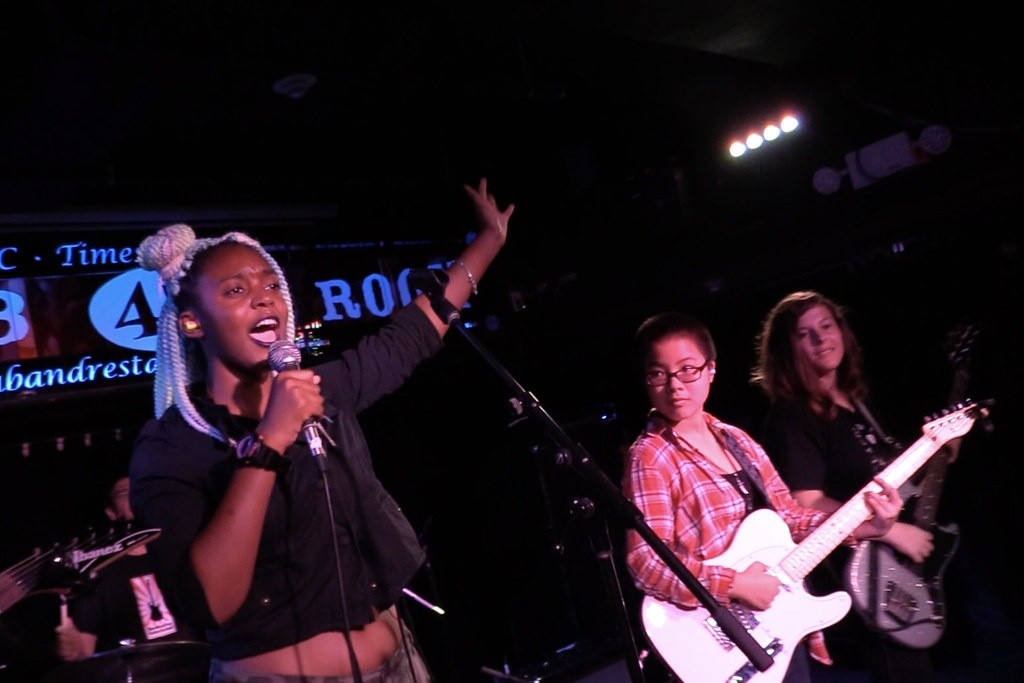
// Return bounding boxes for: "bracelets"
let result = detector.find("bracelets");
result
[455,257,478,294]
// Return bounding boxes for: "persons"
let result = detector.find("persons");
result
[619,309,905,683]
[126,176,515,683]
[56,474,210,661]
[749,290,963,683]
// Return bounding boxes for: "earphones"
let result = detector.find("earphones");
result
[709,369,717,374]
[183,318,199,329]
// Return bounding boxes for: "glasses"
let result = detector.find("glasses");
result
[642,359,710,386]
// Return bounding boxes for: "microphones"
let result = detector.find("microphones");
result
[267,339,328,474]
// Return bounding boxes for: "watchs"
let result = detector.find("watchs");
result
[235,431,292,482]
